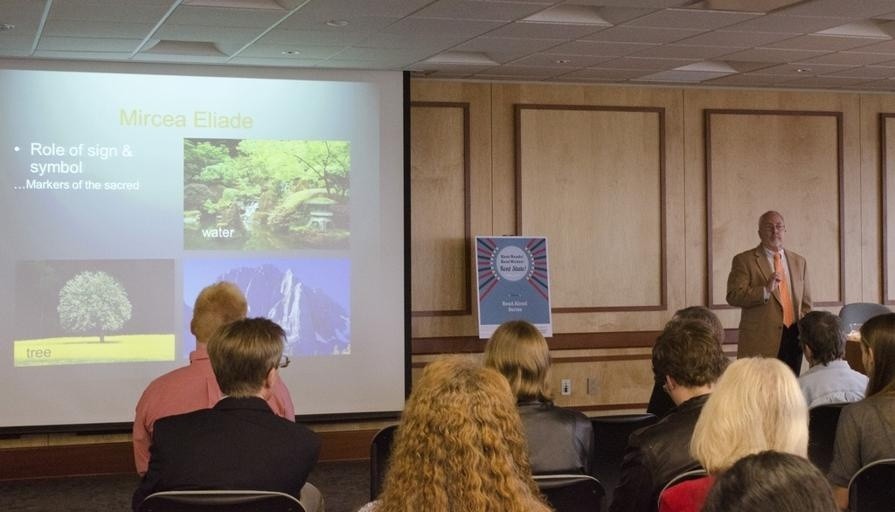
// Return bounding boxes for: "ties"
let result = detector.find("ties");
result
[774,254,794,328]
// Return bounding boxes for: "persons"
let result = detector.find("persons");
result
[133,317,320,511]
[795,309,871,409]
[724,206,814,378]
[479,321,596,478]
[827,309,894,510]
[644,306,731,418]
[601,315,822,512]
[703,449,839,512]
[352,352,557,512]
[654,356,812,512]
[133,277,299,483]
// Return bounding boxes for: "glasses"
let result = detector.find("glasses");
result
[276,356,288,371]
